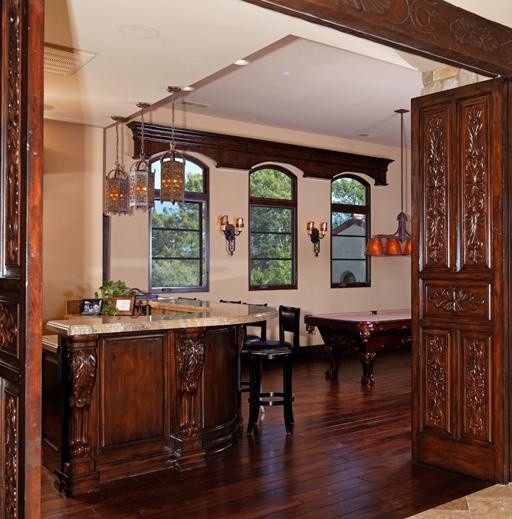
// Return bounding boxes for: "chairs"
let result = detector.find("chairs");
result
[241,303,278,368]
[241,303,301,434]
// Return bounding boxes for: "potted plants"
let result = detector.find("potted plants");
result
[98,279,130,318]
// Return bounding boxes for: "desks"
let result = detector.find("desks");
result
[304,308,412,387]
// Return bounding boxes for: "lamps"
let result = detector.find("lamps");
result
[160,97,185,206]
[236,217,244,234]
[321,221,328,237]
[220,214,229,231]
[128,113,156,217]
[306,220,314,236]
[104,123,129,217]
[364,108,413,258]
